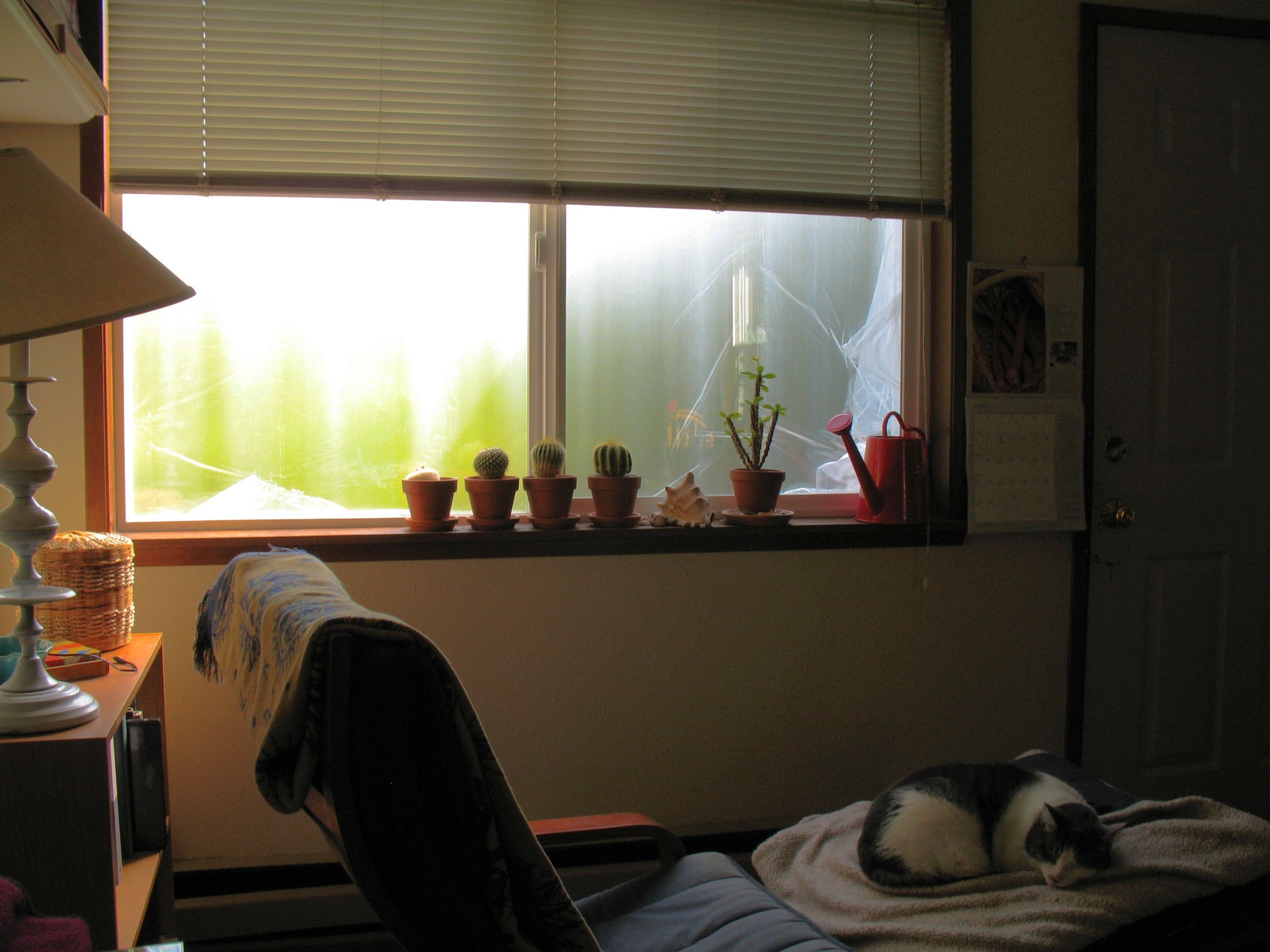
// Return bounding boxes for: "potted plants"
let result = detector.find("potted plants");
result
[522,437,581,530]
[588,442,642,527]
[718,354,796,529]
[402,466,459,531]
[463,446,524,530]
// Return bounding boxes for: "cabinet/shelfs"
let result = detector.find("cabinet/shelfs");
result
[0,632,169,952]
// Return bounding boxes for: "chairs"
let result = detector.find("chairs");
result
[191,550,852,952]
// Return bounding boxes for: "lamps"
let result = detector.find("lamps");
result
[0,146,196,737]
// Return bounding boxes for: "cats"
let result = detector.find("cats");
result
[857,761,1129,889]
